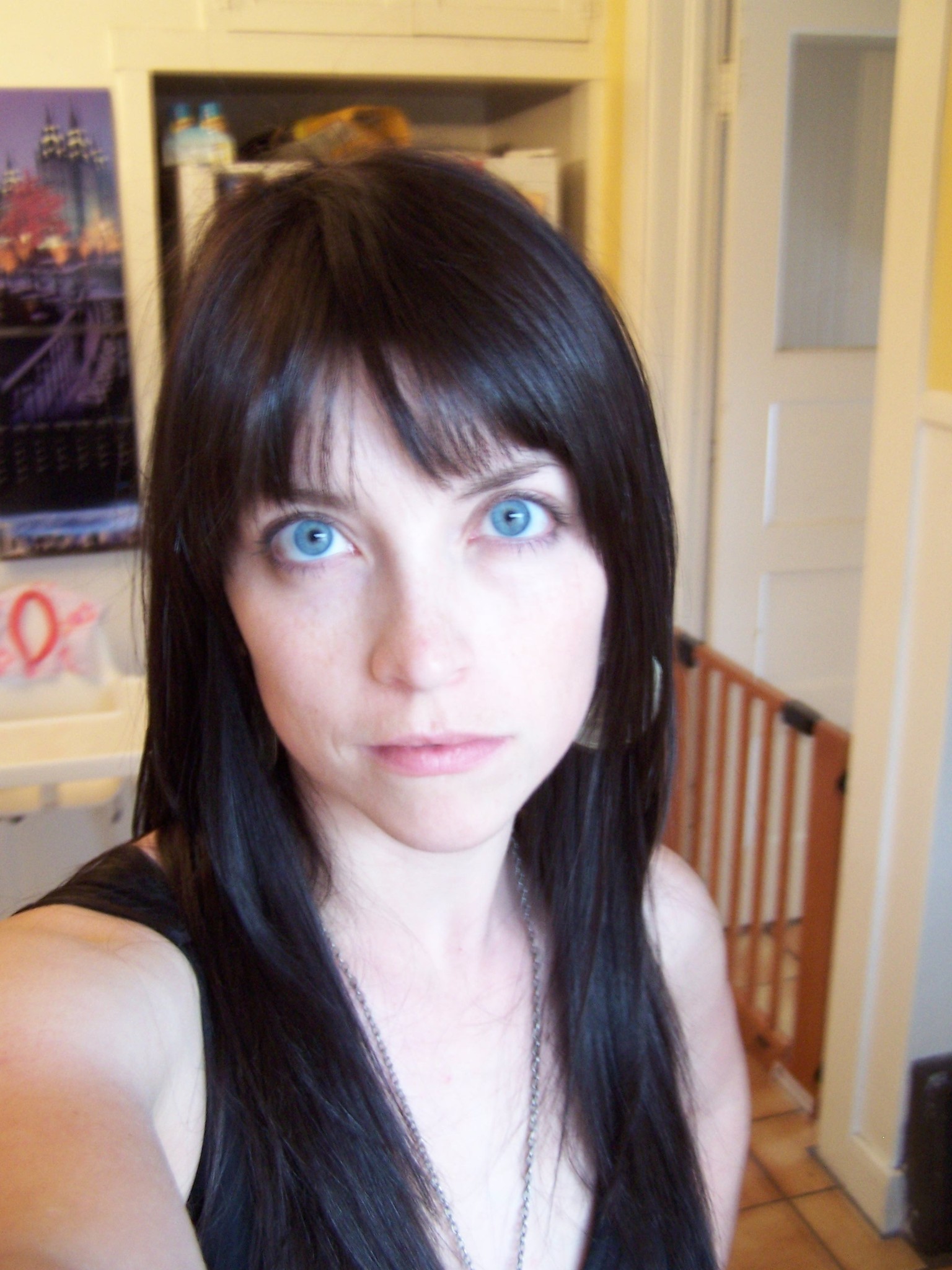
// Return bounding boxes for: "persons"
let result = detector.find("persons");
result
[0,143,755,1270]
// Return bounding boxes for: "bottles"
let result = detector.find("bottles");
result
[159,100,236,166]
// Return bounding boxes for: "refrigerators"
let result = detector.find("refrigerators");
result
[159,146,563,278]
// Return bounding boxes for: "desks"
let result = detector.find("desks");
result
[0,670,150,916]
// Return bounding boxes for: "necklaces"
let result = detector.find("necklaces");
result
[304,838,542,1270]
